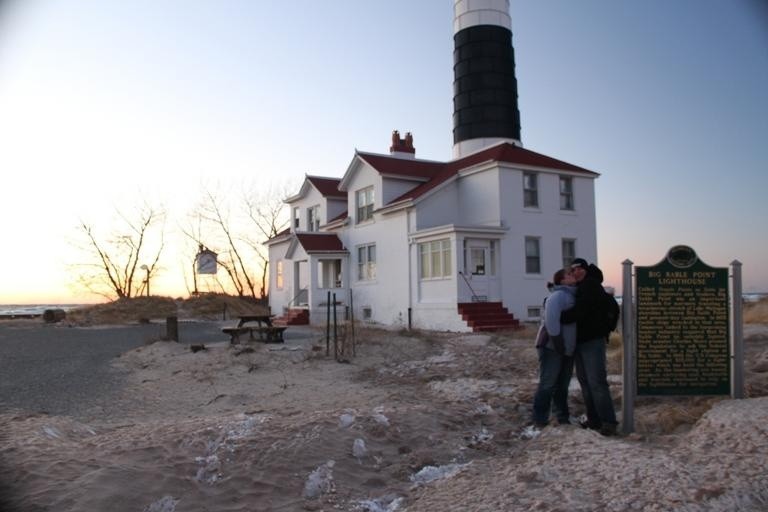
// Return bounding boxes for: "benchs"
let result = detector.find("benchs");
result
[222,326,248,344]
[261,327,286,345]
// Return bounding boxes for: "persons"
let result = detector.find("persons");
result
[541,257,621,437]
[530,268,579,429]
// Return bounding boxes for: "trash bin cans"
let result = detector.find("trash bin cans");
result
[167,317,178,342]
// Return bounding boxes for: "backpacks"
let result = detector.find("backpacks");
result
[603,290,621,333]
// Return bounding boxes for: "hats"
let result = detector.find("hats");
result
[570,257,589,271]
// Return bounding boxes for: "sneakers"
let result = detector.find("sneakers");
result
[598,422,618,437]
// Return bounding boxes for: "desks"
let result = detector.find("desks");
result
[222,314,287,347]
